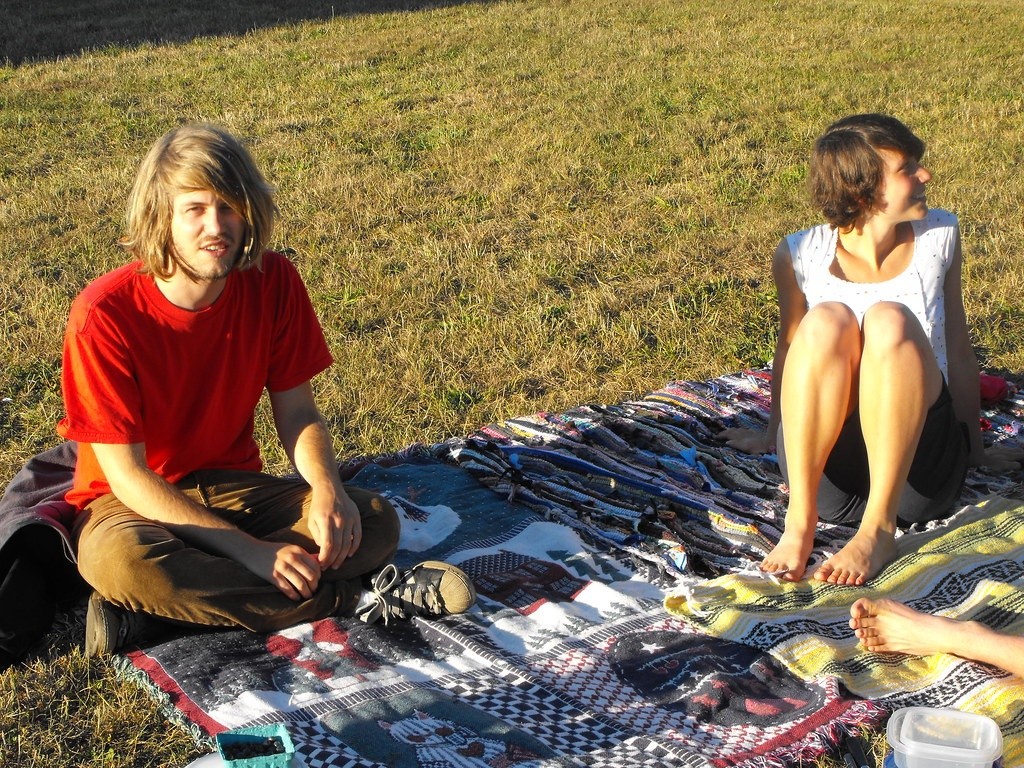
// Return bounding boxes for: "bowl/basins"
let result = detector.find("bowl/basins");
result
[881,706,1005,768]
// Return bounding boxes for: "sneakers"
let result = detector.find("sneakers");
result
[85,590,164,657]
[355,560,476,618]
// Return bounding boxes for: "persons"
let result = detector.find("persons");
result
[61,125,477,660]
[715,112,1024,585]
[846,598,1024,687]
